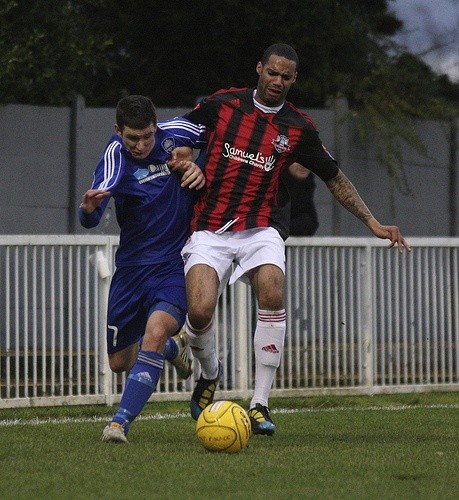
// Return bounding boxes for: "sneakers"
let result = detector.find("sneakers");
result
[171,329,193,379]
[190,360,223,420]
[250,403,276,435]
[102,423,128,442]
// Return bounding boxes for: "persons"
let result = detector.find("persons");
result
[283,161,319,236]
[180,43,412,437]
[78,96,211,444]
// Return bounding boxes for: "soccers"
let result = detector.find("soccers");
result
[195,401,254,454]
